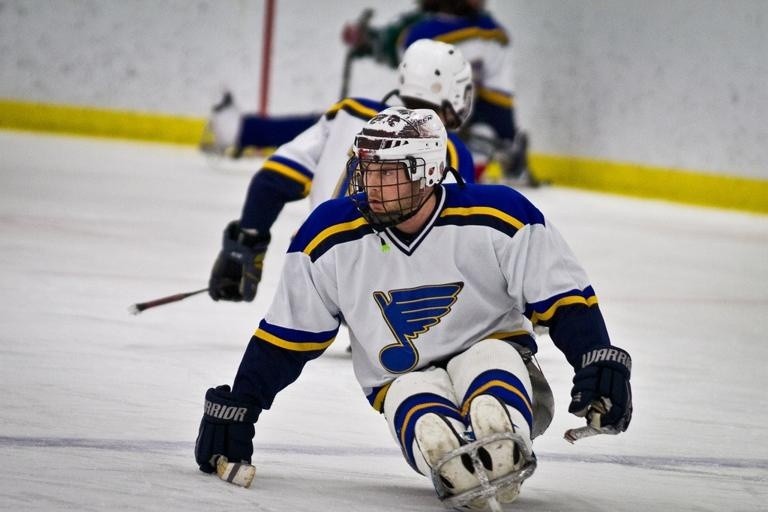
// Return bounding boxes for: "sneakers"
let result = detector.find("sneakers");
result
[411,408,496,512]
[208,86,243,154]
[469,391,534,506]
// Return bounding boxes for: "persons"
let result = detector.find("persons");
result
[203,1,540,187]
[192,107,634,512]
[204,37,478,304]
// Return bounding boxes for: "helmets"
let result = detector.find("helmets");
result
[350,105,450,191]
[395,35,477,116]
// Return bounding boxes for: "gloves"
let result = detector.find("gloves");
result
[343,20,381,61]
[498,131,542,189]
[190,382,264,476]
[206,216,274,305]
[564,344,636,437]
